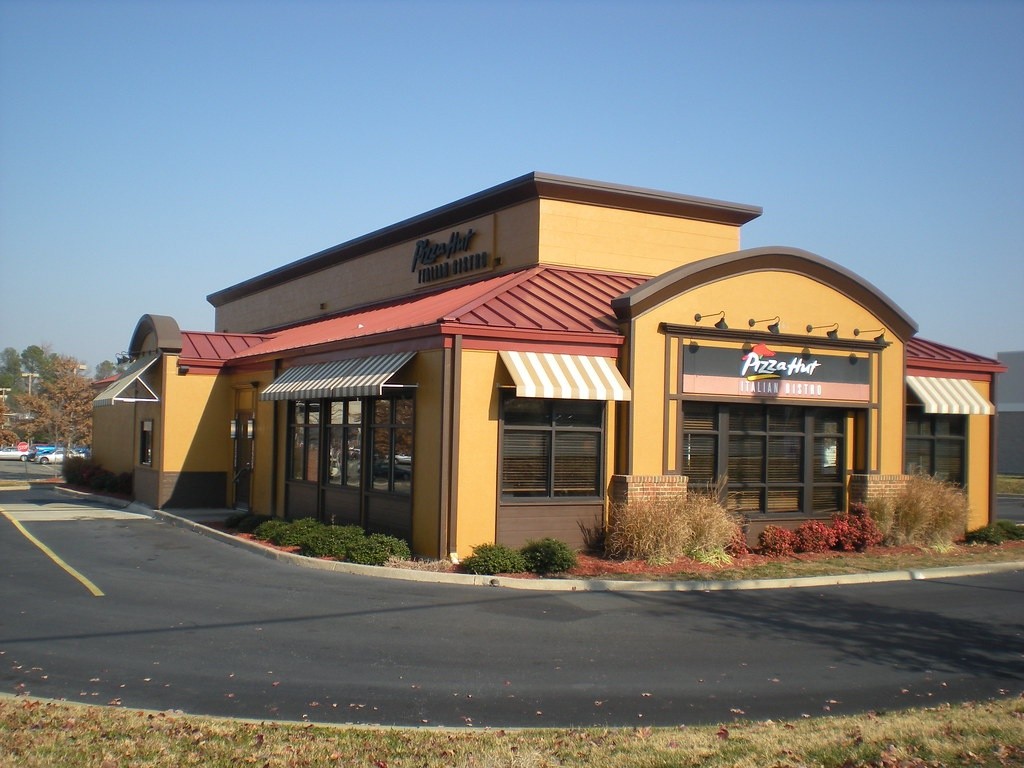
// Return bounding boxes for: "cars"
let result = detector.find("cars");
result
[0,447,33,462]
[30,445,91,460]
[35,449,86,464]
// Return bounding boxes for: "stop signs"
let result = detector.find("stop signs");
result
[18,442,28,453]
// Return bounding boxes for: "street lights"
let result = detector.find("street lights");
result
[22,372,40,395]
[64,363,87,389]
[0,388,12,403]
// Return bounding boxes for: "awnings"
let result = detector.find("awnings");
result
[92,349,163,407]
[258,350,417,401]
[905,376,997,415]
[498,351,633,401]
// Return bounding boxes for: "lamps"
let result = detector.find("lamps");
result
[853,328,887,346]
[121,350,151,363]
[230,419,238,440]
[694,310,728,329]
[806,322,839,341]
[128,348,158,364]
[749,316,780,334]
[115,352,140,365]
[247,419,255,440]
